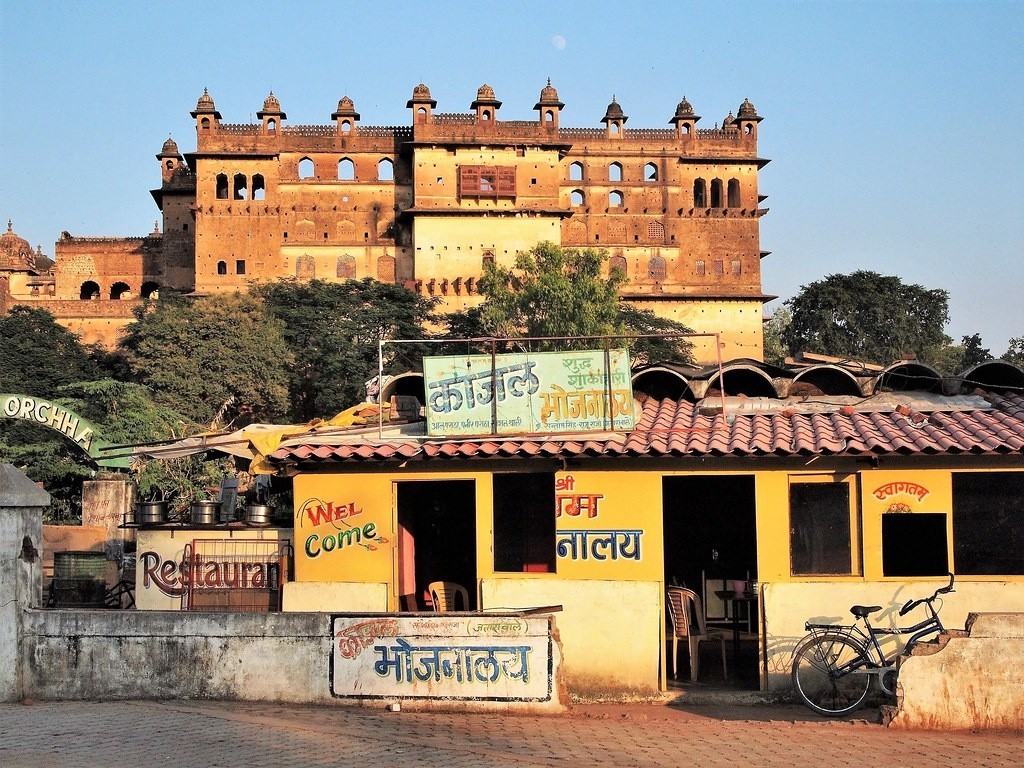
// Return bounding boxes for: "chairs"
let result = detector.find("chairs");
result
[665,584,729,682]
[428,581,469,612]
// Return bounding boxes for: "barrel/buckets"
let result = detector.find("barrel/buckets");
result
[52,550,106,608]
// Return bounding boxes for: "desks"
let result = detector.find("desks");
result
[715,590,757,670]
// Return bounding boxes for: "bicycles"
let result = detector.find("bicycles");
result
[790,571,955,717]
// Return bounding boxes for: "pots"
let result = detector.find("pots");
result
[242,503,278,524]
[135,501,169,524]
[190,500,224,525]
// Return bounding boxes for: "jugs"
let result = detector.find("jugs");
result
[732,580,748,598]
